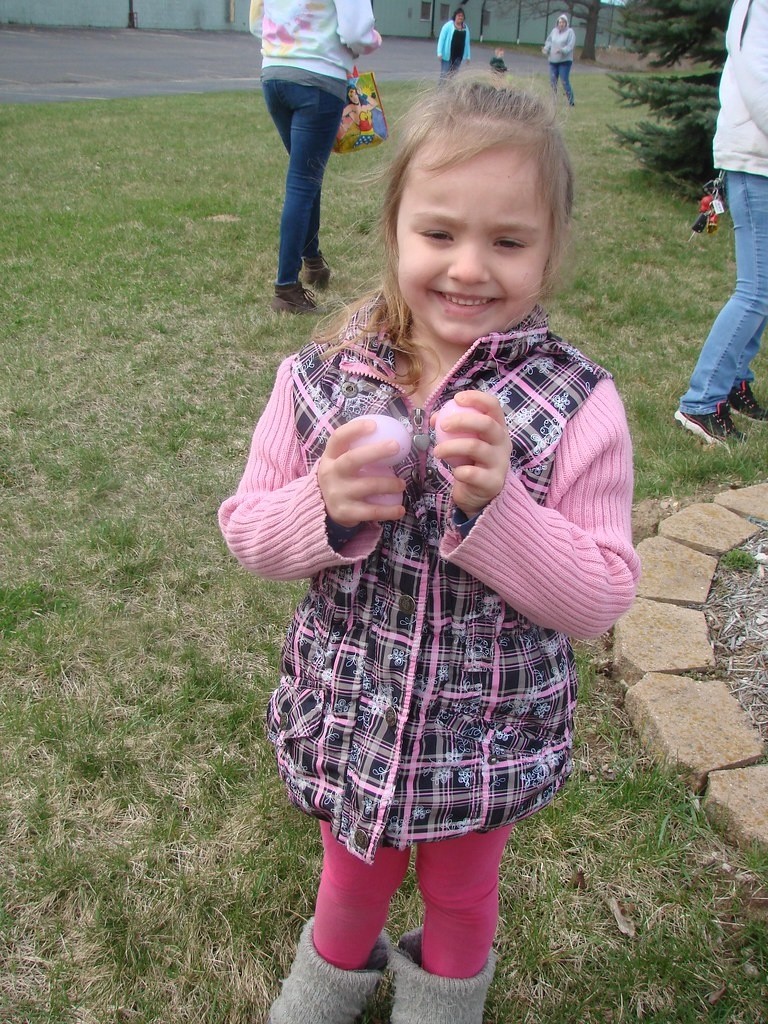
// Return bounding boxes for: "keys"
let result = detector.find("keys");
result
[686,179,725,247]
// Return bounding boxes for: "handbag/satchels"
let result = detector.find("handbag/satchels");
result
[331,65,390,154]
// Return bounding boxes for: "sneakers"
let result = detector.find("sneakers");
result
[728,381,768,425]
[674,401,747,446]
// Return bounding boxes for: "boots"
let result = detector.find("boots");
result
[267,917,392,1023]
[386,927,497,1023]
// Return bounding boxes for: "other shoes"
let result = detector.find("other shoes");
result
[273,282,328,315]
[302,252,329,284]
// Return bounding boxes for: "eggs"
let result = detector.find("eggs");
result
[347,399,487,506]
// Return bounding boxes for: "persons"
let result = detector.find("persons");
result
[214,79,641,1024]
[541,14,578,108]
[488,48,510,77]
[248,0,381,317]
[672,1,768,445]
[436,9,473,89]
